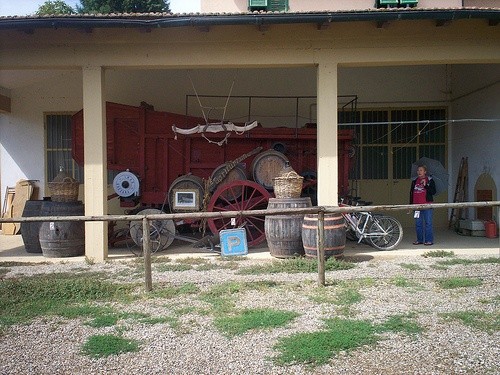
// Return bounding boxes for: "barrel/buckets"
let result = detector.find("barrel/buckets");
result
[485,219,496,238]
[301,212,346,259]
[38,221,85,258]
[264,197,312,257]
[130,208,176,251]
[210,163,248,202]
[168,174,207,214]
[250,149,290,190]
[20,200,85,253]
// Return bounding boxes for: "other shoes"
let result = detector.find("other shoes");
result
[413,241,421,244]
[424,242,433,245]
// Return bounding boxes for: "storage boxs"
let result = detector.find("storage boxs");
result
[460,220,485,237]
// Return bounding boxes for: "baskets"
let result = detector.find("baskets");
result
[48,177,79,203]
[272,172,304,199]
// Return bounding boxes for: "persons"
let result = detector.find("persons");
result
[408,165,437,245]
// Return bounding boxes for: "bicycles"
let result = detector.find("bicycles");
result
[337,195,404,251]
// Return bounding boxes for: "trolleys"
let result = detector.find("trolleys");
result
[106,176,317,258]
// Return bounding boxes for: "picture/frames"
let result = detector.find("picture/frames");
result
[173,189,199,210]
